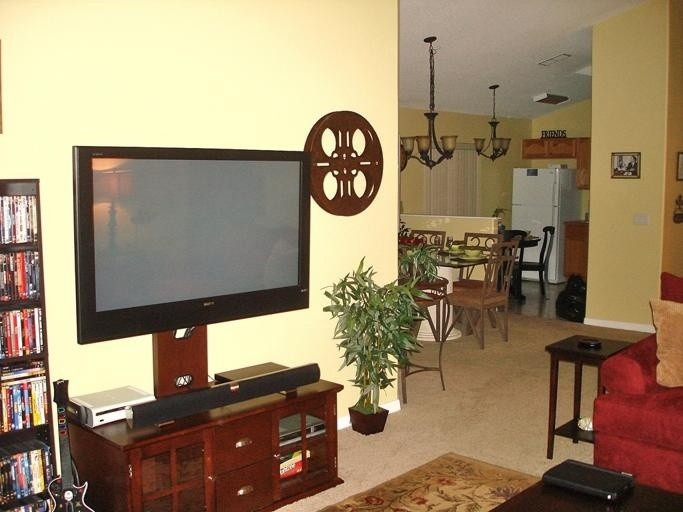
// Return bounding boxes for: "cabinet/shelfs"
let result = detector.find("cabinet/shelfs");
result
[1,180,57,512]
[66,362,344,512]
[564,222,588,279]
[522,139,576,157]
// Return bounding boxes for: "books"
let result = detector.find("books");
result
[1,194,51,511]
[280,450,311,479]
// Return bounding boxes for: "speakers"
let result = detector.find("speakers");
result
[125,362,321,432]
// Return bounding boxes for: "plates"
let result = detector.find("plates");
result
[459,256,486,261]
[449,251,464,255]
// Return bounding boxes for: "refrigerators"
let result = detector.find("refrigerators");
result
[512,167,581,283]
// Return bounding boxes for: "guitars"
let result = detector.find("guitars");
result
[49,379,97,512]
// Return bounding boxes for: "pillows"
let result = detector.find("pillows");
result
[649,297,683,387]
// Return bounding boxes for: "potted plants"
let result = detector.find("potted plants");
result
[320,255,434,436]
[400,242,442,282]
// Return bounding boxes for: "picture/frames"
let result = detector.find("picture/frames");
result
[610,151,641,178]
[677,152,683,181]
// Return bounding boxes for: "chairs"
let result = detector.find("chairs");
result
[510,225,556,299]
[498,230,526,299]
[411,230,446,248]
[414,292,442,342]
[442,240,520,350]
[453,232,504,334]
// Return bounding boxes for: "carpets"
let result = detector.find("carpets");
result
[316,451,541,512]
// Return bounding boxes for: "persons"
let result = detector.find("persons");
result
[614,154,638,176]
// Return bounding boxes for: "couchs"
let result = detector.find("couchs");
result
[593,272,683,493]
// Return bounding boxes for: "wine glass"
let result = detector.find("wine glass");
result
[447,237,453,259]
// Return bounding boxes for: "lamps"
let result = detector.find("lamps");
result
[472,84,512,163]
[533,94,569,105]
[400,36,458,169]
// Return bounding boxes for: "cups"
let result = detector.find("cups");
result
[451,245,462,250]
[464,249,482,257]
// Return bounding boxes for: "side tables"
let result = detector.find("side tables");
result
[545,334,635,459]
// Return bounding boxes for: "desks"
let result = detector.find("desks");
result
[513,239,541,248]
[400,277,449,404]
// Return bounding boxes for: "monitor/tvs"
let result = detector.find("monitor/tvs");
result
[71,145,309,346]
